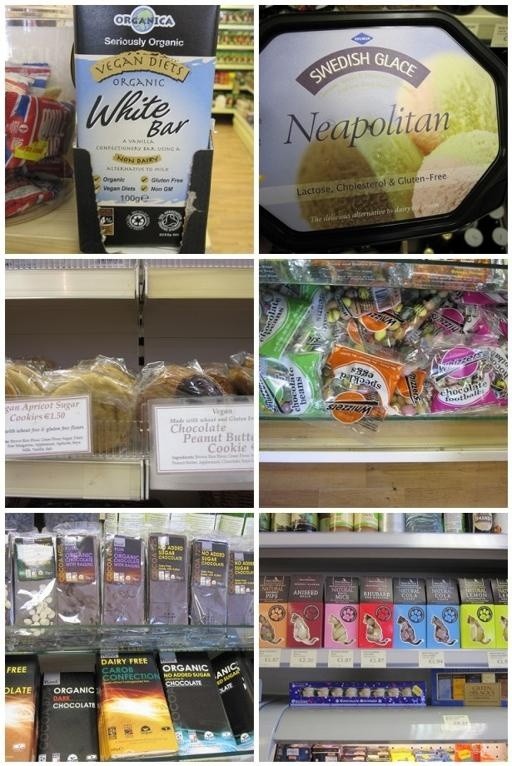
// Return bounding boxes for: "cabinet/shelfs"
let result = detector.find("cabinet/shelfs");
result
[259,533,507,760]
[5,623,254,761]
[211,7,254,117]
[232,82,254,161]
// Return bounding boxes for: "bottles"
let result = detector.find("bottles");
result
[258,513,509,535]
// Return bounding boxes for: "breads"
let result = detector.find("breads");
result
[7,351,252,453]
